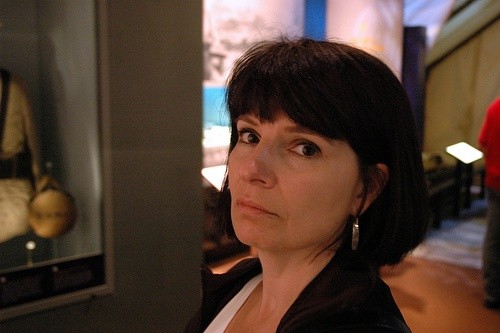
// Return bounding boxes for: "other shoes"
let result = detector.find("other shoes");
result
[484,295,500,310]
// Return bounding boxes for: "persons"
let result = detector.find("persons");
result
[475,98,500,312]
[185,40,427,333]
[0,67,44,243]
